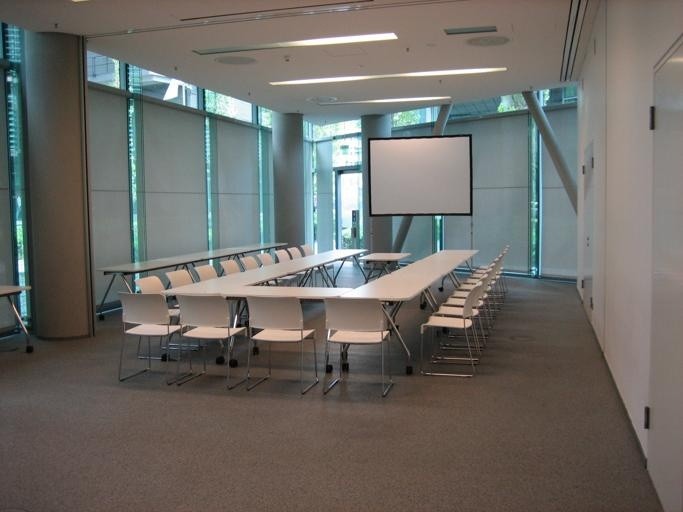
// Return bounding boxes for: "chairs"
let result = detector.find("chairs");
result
[431,244,512,362]
[321,297,395,398]
[243,294,320,396]
[115,290,187,386]
[133,241,337,350]
[172,293,248,390]
[417,280,483,378]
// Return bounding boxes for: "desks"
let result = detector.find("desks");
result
[159,247,368,374]
[339,247,482,375]
[94,243,286,323]
[358,249,411,284]
[2,284,35,354]
